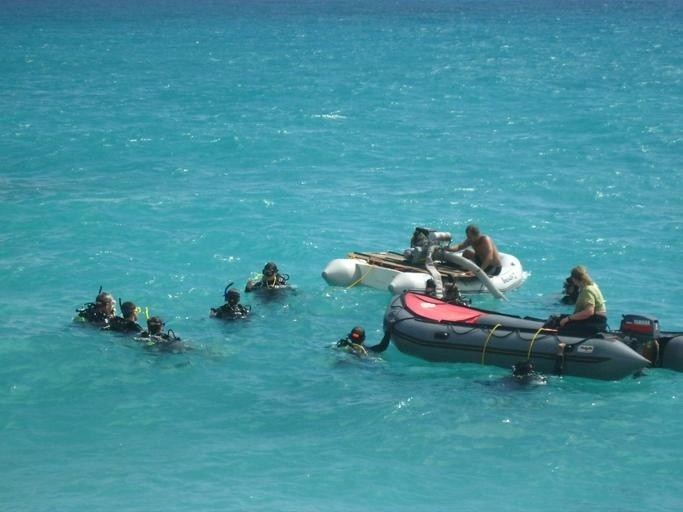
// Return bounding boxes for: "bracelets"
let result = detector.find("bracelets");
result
[567,315,571,321]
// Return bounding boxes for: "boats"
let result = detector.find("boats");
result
[321,248,525,302]
[385,290,682,382]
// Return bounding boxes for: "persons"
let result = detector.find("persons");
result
[539,264,607,337]
[99,297,144,339]
[474,358,548,394]
[245,262,298,306]
[551,276,578,306]
[323,320,390,372]
[73,285,115,327]
[209,282,252,334]
[442,225,502,282]
[131,306,185,354]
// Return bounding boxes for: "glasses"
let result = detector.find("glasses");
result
[262,269,273,276]
